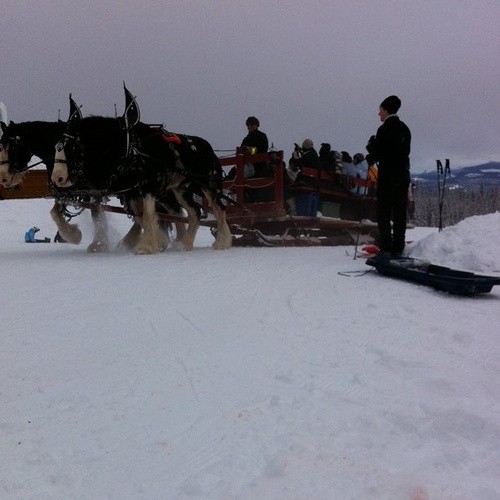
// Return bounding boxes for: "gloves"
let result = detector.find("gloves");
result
[365,153,375,167]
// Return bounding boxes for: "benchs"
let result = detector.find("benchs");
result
[292,166,418,210]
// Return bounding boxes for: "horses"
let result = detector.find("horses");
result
[0,78,235,255]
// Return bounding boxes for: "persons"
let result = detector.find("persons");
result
[366,95,412,255]
[291,139,378,194]
[54,231,67,243]
[25,226,51,243]
[224,117,269,180]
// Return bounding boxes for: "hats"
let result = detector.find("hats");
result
[246,116,259,126]
[380,95,401,115]
[302,137,313,149]
